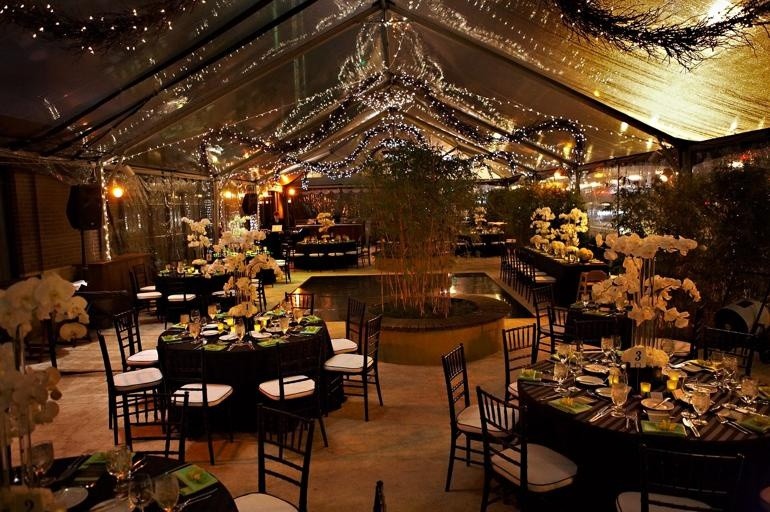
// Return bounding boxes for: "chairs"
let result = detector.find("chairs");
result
[249,214,374,286]
[156,346,236,466]
[373,479,387,511]
[643,306,703,364]
[530,284,635,366]
[258,337,330,460]
[112,307,159,372]
[119,389,190,463]
[701,325,759,383]
[329,297,365,356]
[323,314,384,422]
[500,322,541,411]
[614,442,746,512]
[284,291,315,317]
[232,403,317,511]
[475,386,579,512]
[93,328,165,447]
[441,342,521,493]
[127,250,267,330]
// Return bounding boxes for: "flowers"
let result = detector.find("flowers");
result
[527,205,595,262]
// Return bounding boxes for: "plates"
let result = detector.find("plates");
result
[90,497,135,511]
[52,486,88,509]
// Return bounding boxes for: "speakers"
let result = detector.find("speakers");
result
[241,193,258,215]
[66,184,103,231]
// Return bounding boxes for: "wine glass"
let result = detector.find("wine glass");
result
[528,336,770,439]
[305,235,349,243]
[21,440,56,486]
[167,301,319,351]
[166,244,267,277]
[533,241,627,314]
[105,443,179,512]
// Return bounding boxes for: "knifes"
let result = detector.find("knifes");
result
[57,453,91,480]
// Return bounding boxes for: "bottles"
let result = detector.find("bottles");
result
[331,207,361,224]
